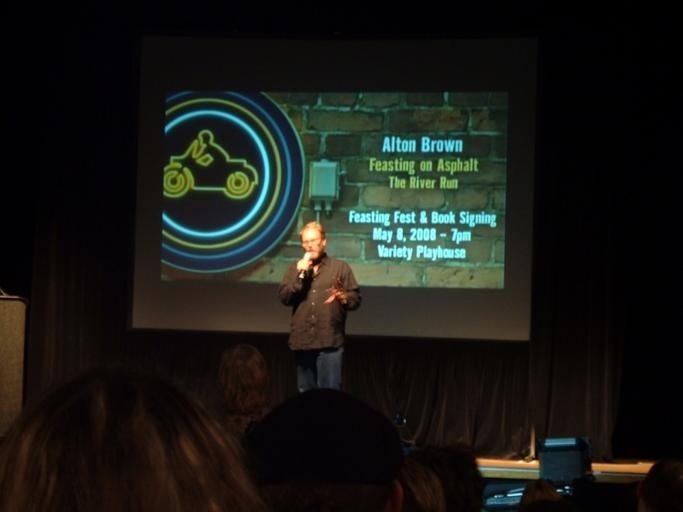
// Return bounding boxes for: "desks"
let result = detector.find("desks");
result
[476,456,657,512]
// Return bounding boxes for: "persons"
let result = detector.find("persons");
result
[412,440,486,512]
[519,476,561,504]
[238,386,409,512]
[636,459,683,511]
[197,338,272,441]
[0,368,269,512]
[277,222,362,390]
[402,463,447,511]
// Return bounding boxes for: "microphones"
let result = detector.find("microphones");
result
[298,252,311,279]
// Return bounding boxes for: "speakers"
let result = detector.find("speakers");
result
[0,296,29,437]
